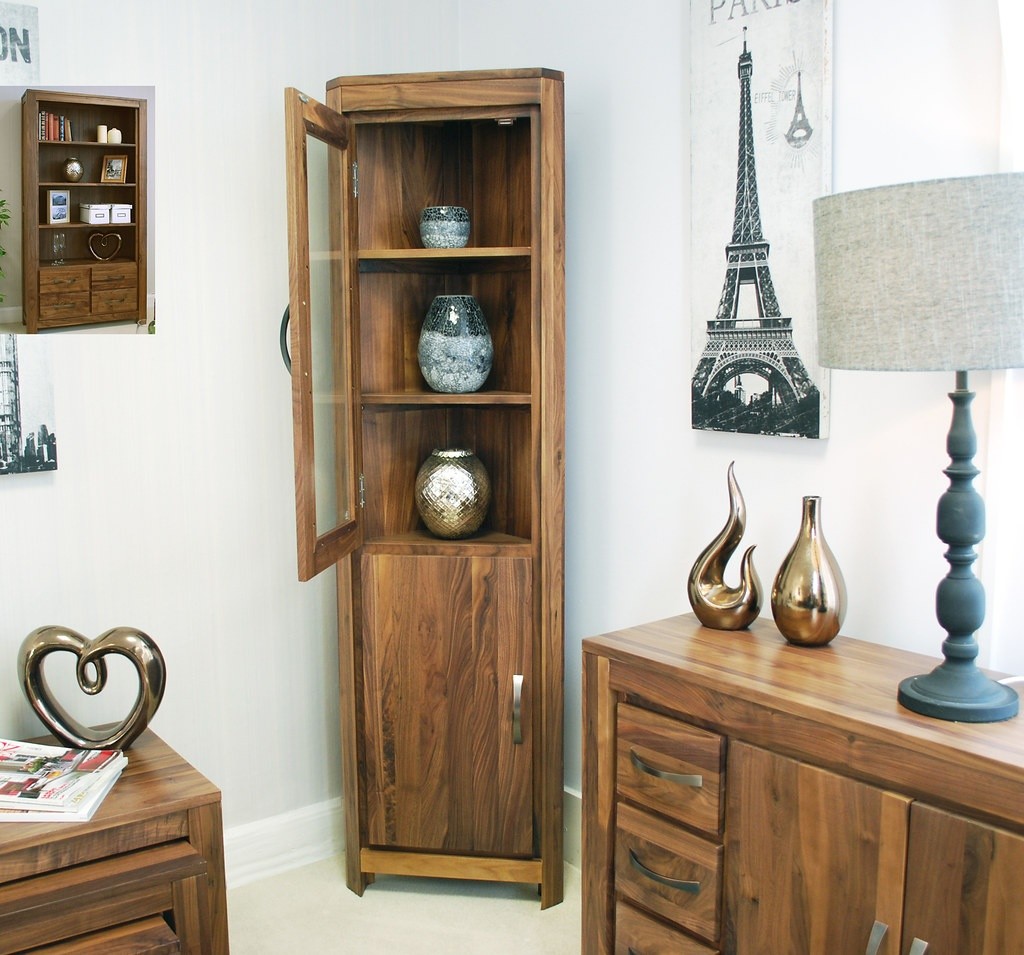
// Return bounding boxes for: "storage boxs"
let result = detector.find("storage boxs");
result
[79,203,132,224]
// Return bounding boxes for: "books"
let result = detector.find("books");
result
[39,111,72,141]
[0,738,128,823]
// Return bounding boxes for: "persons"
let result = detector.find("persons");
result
[107,164,113,178]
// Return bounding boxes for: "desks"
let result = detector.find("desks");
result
[0,720,229,955]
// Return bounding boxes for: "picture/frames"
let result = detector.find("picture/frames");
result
[47,190,71,225]
[100,155,127,184]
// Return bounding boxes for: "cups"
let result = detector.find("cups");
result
[50,233,65,266]
[97,125,107,143]
[419,206,470,248]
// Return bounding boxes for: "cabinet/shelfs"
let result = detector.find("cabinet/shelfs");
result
[20,89,148,334]
[579,612,1024,955]
[284,68,566,910]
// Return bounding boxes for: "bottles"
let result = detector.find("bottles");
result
[416,294,494,394]
[771,496,848,648]
[108,128,122,144]
[62,157,84,182]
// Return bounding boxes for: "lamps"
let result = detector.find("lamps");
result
[814,170,1024,724]
